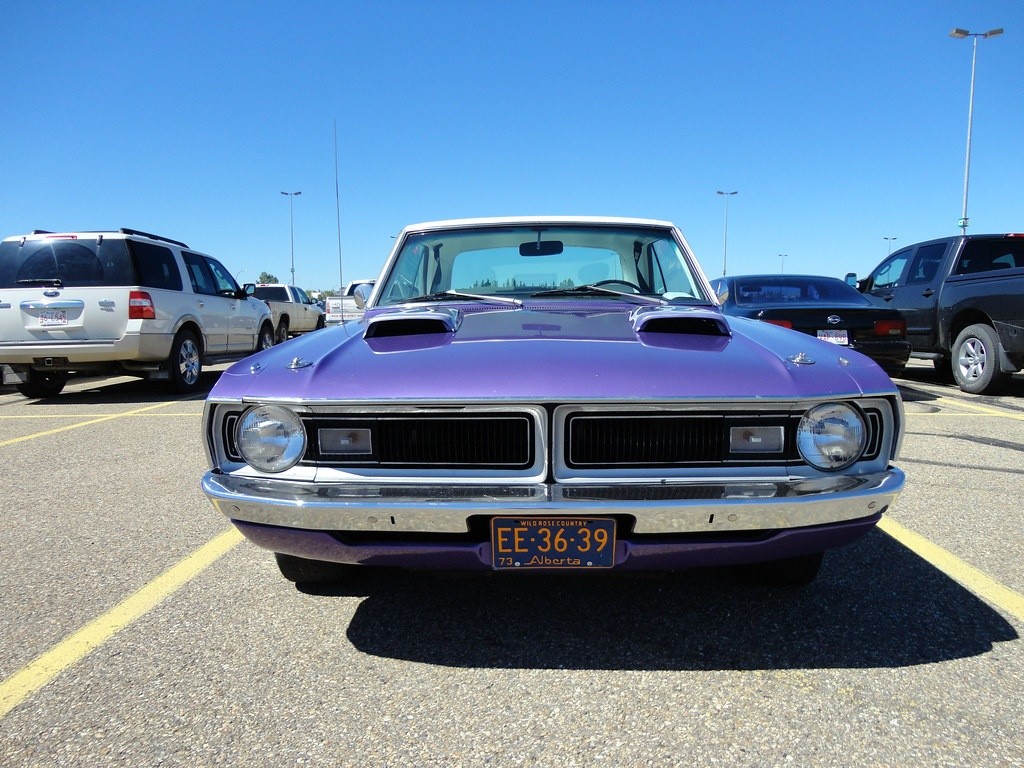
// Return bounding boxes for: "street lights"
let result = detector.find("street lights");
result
[779,255,787,274]
[948,25,1005,234]
[884,236,897,282]
[281,192,303,285]
[716,190,738,276]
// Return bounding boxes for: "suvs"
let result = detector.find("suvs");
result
[0,229,275,399]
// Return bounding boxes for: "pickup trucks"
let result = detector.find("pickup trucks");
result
[845,233,1023,395]
[242,284,325,345]
[323,279,411,330]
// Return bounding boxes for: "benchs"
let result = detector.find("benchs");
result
[968,261,1012,271]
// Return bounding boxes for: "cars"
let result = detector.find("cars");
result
[690,274,911,380]
[200,214,905,586]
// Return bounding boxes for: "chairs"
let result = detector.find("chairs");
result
[915,261,940,282]
[129,253,166,289]
